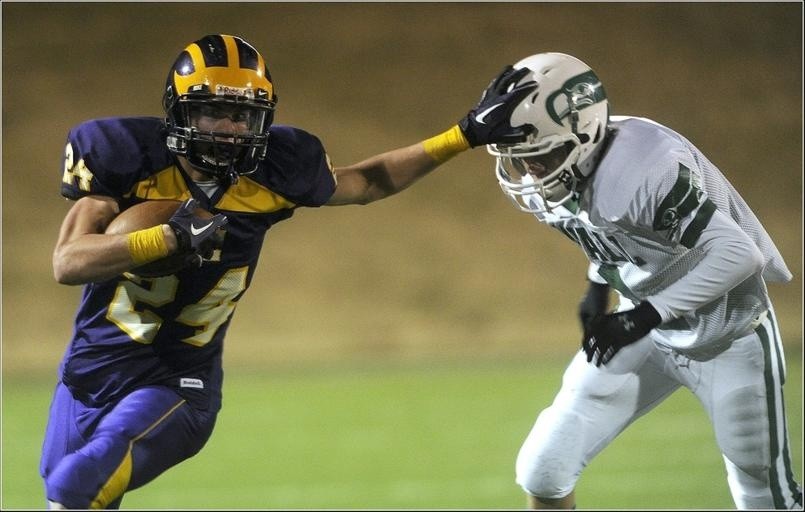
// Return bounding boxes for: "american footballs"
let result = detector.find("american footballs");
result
[104,199,220,277]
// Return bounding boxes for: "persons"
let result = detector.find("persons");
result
[486,52,802,510]
[39,35,539,509]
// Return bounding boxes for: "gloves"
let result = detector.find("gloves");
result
[167,198,229,255]
[457,66,537,149]
[579,282,661,367]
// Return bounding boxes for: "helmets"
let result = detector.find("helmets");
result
[485,52,610,192]
[166,34,274,109]
